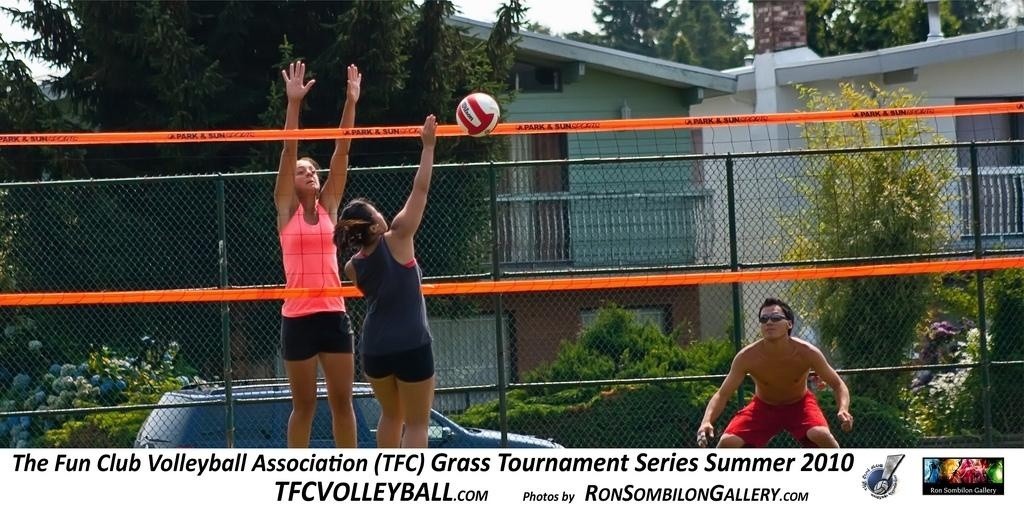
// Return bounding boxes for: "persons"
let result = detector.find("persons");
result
[274,60,362,448]
[696,299,854,448]
[333,114,438,448]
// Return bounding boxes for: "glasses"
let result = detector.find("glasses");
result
[758,313,787,323]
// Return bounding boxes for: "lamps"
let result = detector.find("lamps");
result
[620,97,632,120]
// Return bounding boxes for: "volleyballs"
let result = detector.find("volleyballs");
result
[456,93,500,138]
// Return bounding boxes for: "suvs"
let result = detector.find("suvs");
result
[123,373,569,448]
